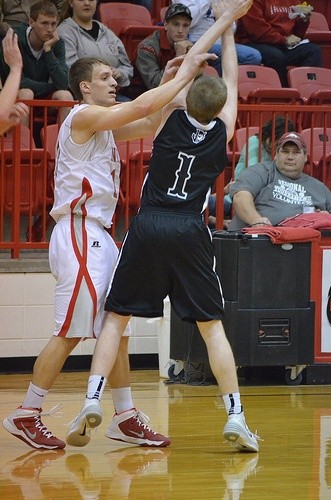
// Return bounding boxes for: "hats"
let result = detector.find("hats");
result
[164,2,193,22]
[277,131,306,152]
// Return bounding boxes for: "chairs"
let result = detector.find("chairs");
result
[0,0,331,257]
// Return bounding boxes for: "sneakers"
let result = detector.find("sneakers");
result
[104,407,172,448]
[221,413,260,451]
[65,404,104,446]
[2,405,66,451]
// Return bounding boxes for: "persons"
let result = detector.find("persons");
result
[170,0,323,87]
[2,58,172,451]
[67,0,261,454]
[135,4,193,90]
[0,0,133,148]
[229,132,331,232]
[208,115,295,230]
